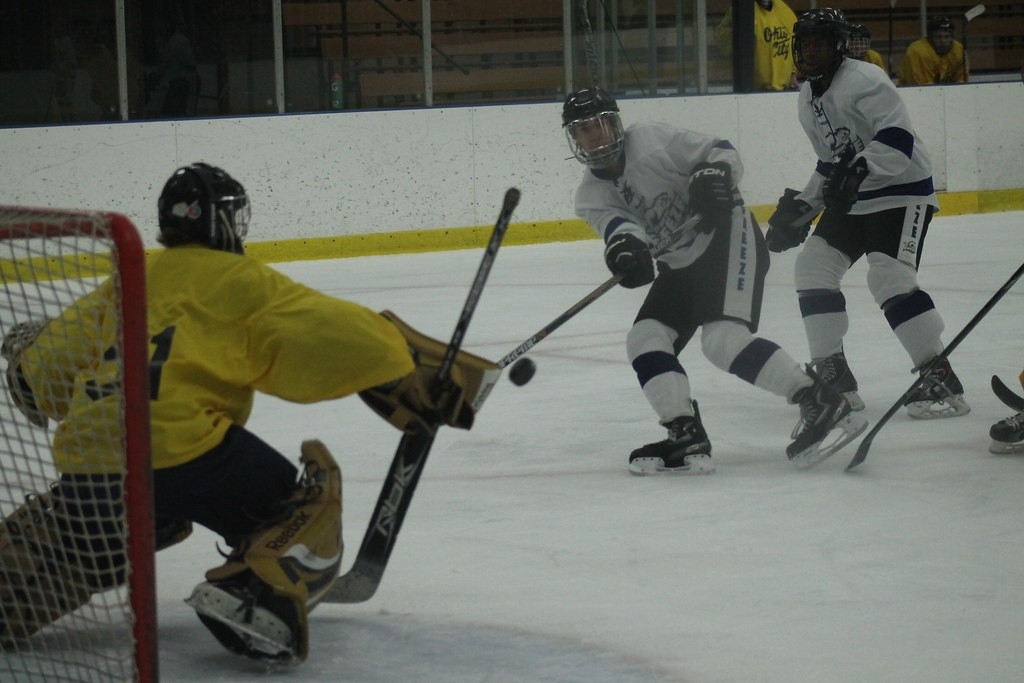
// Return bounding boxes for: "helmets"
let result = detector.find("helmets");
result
[562,87,626,169]
[849,23,871,58]
[789,8,851,82]
[157,163,252,251]
[928,17,955,54]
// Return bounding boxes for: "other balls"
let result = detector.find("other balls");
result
[509,358,536,387]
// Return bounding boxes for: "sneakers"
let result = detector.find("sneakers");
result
[988,412,1024,454]
[815,339,866,411]
[784,379,869,466]
[903,357,971,420]
[188,574,293,656]
[628,400,717,476]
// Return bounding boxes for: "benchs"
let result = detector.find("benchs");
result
[281,0,1024,108]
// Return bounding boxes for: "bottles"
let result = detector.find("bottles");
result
[415,92,440,102]
[790,72,799,91]
[330,73,343,111]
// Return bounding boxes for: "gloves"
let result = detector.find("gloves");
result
[765,187,812,253]
[604,233,658,291]
[686,161,737,235]
[822,156,870,216]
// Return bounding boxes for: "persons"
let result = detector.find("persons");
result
[848,24,887,76]
[768,9,970,420]
[0,163,460,662]
[904,19,968,85]
[989,372,1024,453]
[562,89,867,473]
[716,0,798,94]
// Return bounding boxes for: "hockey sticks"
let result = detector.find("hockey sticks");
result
[845,262,1024,470]
[766,191,859,246]
[495,213,705,371]
[318,184,523,607]
[991,374,1023,413]
